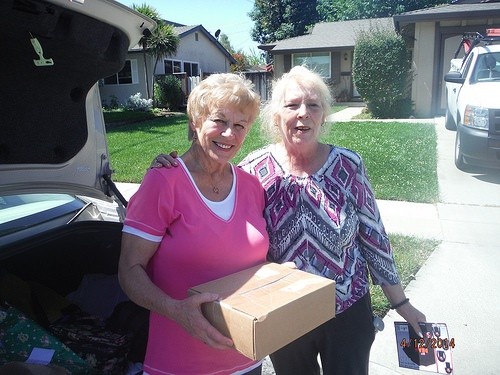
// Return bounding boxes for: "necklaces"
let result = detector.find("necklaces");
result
[188,149,223,194]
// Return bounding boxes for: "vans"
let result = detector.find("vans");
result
[0,0,159,205]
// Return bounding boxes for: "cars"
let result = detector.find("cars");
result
[442,28,500,173]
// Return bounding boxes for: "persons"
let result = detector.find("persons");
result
[152,66,427,375]
[117,73,297,375]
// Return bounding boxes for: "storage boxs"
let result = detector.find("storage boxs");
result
[188,262,336,361]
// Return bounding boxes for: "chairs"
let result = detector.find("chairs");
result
[477,56,500,79]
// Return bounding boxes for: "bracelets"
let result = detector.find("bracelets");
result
[389,298,409,310]
